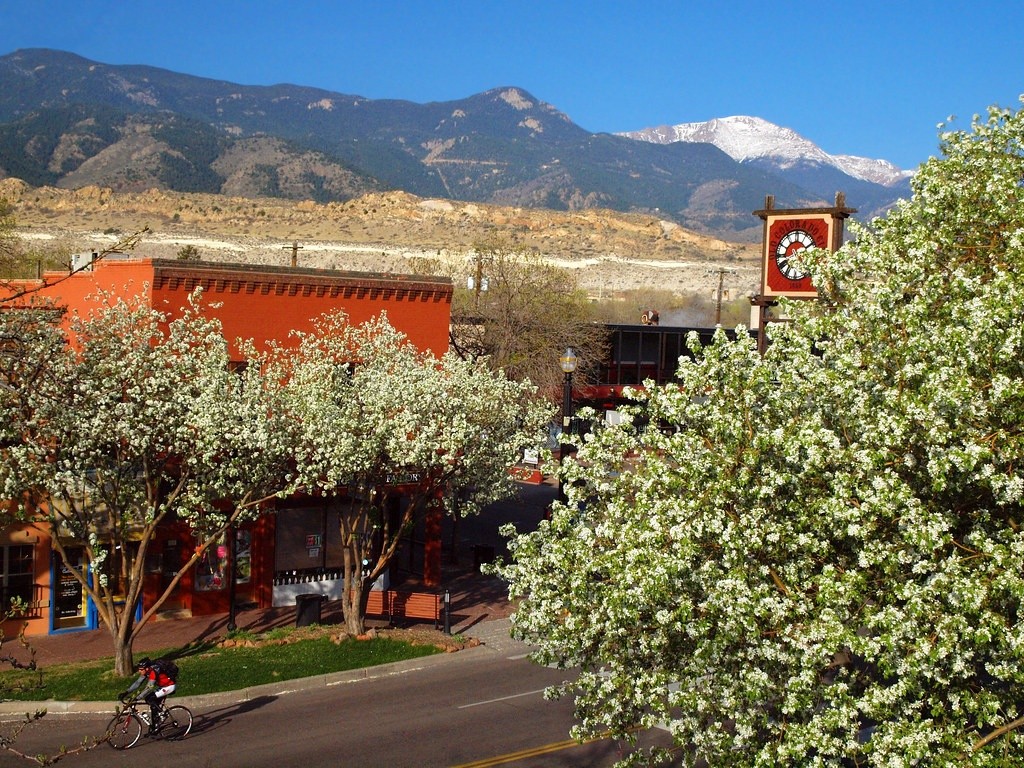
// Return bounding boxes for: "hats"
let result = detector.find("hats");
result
[136,658,151,667]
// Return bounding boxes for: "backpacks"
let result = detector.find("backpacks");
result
[144,657,179,679]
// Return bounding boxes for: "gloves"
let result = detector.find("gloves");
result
[118,691,129,701]
[129,697,138,703]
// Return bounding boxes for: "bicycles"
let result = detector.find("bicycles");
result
[105,696,194,751]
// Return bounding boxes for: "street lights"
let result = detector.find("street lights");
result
[559,348,578,505]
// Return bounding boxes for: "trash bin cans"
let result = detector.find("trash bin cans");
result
[295,594,323,627]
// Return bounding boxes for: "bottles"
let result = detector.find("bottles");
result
[142,711,151,723]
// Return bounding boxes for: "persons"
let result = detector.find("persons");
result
[118,657,175,736]
